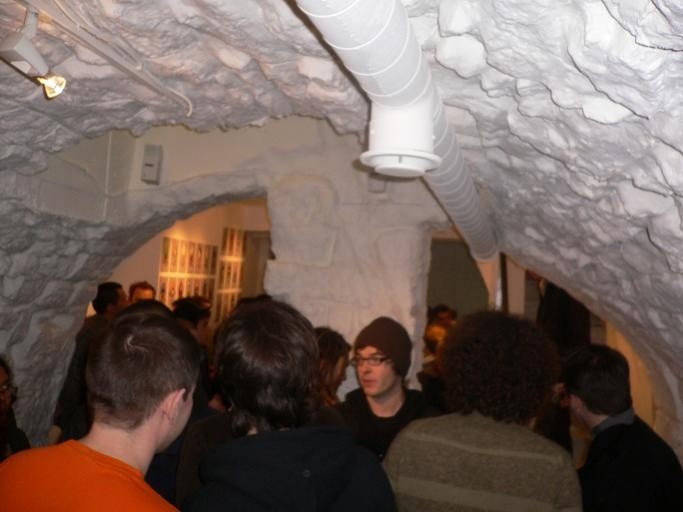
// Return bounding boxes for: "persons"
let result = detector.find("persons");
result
[0,310,206,512]
[421,305,458,375]
[50,280,220,446]
[562,344,683,511]
[313,316,445,463]
[381,310,583,511]
[0,355,32,461]
[145,300,400,511]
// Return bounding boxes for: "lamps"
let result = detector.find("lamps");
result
[0,7,67,99]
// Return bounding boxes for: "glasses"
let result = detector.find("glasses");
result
[351,354,390,366]
